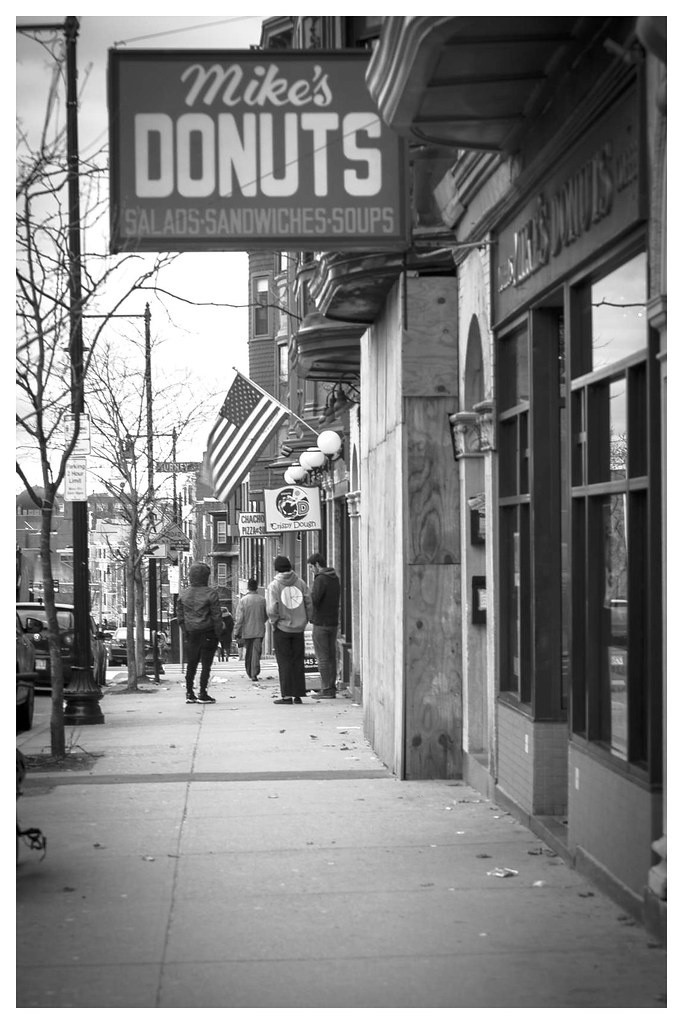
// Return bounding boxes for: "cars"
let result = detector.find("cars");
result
[16,611,44,731]
[110,627,150,664]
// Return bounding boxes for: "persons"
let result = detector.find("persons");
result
[306,553,341,699]
[220,607,234,662]
[266,555,314,705]
[234,578,269,681]
[177,562,222,704]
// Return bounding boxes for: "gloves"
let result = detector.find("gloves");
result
[234,634,241,640]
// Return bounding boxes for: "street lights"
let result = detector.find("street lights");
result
[121,428,183,663]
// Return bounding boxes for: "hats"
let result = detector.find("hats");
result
[275,556,291,571]
[248,578,257,590]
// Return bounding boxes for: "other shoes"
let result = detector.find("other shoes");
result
[247,672,251,678]
[199,694,215,703]
[273,698,292,704]
[311,687,336,699]
[185,692,199,702]
[294,697,301,704]
[252,676,258,681]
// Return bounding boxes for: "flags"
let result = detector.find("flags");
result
[196,373,291,505]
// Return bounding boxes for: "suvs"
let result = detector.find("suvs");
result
[16,601,107,693]
